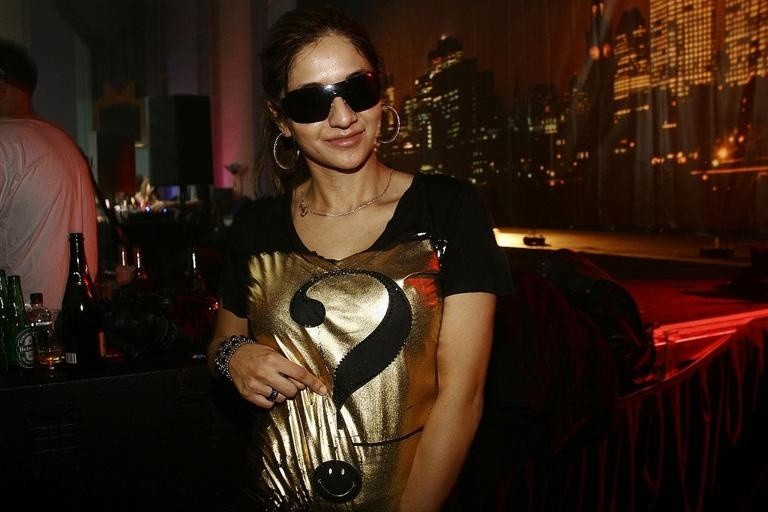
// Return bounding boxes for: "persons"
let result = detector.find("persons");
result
[207,2,514,512]
[0,36,100,315]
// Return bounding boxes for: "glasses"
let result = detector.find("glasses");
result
[277,67,383,125]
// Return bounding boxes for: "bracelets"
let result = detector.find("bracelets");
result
[214,335,258,382]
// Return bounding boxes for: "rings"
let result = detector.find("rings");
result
[266,389,278,401]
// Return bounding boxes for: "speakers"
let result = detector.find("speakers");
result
[147,93,214,187]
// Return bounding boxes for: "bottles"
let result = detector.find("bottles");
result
[0,232,218,381]
[104,192,138,222]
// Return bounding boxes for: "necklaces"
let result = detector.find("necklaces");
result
[299,169,395,217]
